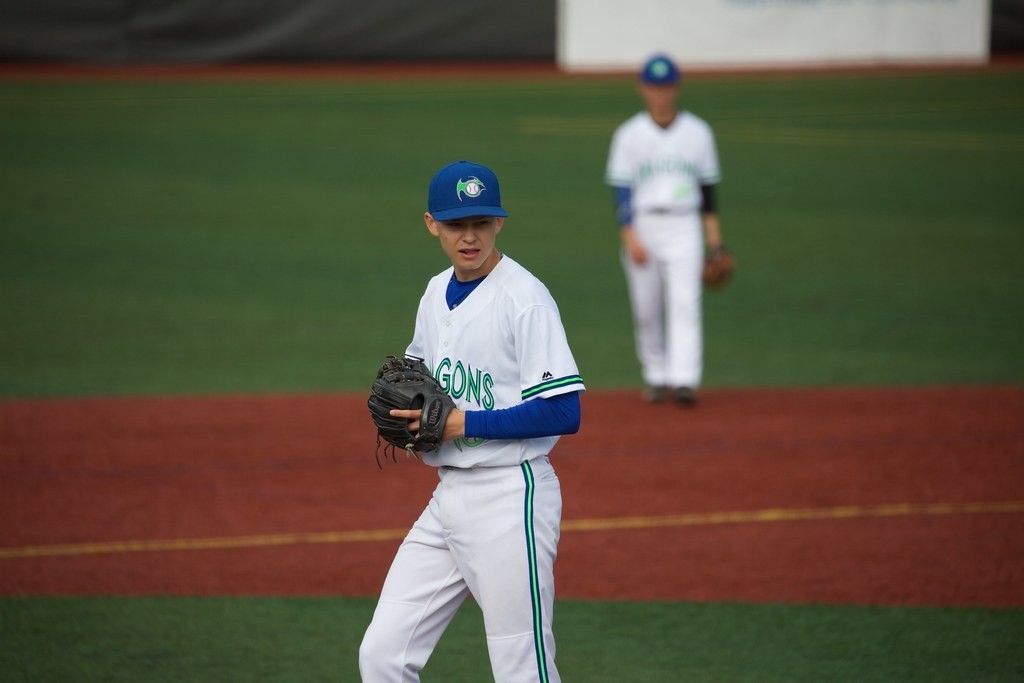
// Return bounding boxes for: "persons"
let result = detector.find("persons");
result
[604,56,725,406]
[357,160,581,683]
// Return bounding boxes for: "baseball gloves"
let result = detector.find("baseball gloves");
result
[704,247,736,291]
[366,352,457,471]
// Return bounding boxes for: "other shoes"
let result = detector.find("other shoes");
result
[676,388,692,403]
[645,386,667,403]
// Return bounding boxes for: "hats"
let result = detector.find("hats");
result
[428,161,508,221]
[642,57,678,84]
